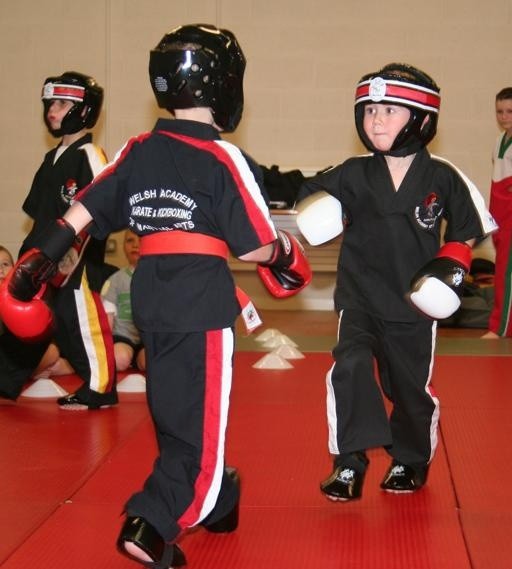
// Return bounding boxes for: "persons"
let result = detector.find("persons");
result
[1,24,314,568]
[100,227,149,373]
[480,86,512,340]
[295,64,499,504]
[1,245,77,380]
[1,71,121,408]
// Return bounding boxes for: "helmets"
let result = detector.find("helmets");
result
[149,24,246,132]
[42,72,103,134]
[354,65,441,156]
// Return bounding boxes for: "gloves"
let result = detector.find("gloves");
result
[405,242,474,320]
[256,229,313,299]
[1,217,76,342]
